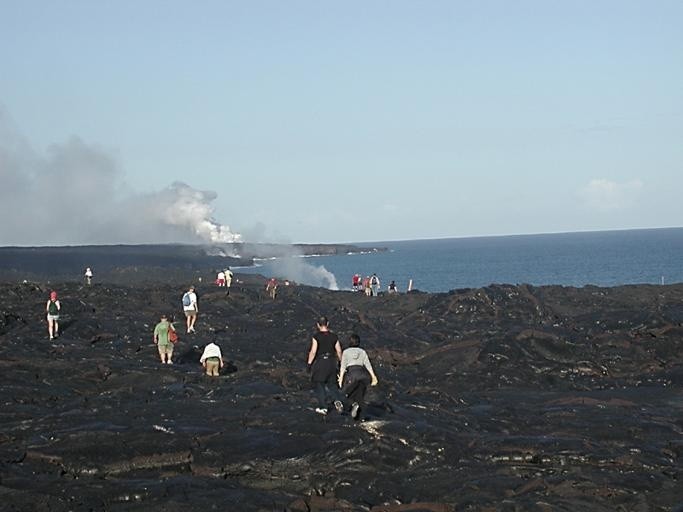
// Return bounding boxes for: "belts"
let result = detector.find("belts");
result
[319,353,334,357]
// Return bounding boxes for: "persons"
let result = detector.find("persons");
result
[181,284,198,334]
[202,342,223,375]
[338,334,377,421]
[216,266,397,300]
[46,292,60,341]
[153,314,175,364]
[84,267,91,286]
[306,315,343,414]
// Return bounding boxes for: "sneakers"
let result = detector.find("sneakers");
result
[162,360,173,364]
[187,327,195,334]
[334,401,344,415]
[351,402,360,418]
[315,408,328,415]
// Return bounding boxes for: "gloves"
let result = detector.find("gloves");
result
[305,365,311,374]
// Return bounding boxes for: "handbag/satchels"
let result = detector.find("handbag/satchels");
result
[167,323,177,344]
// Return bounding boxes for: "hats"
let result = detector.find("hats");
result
[50,291,57,299]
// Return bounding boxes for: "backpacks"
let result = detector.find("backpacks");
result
[182,292,193,306]
[48,300,58,315]
